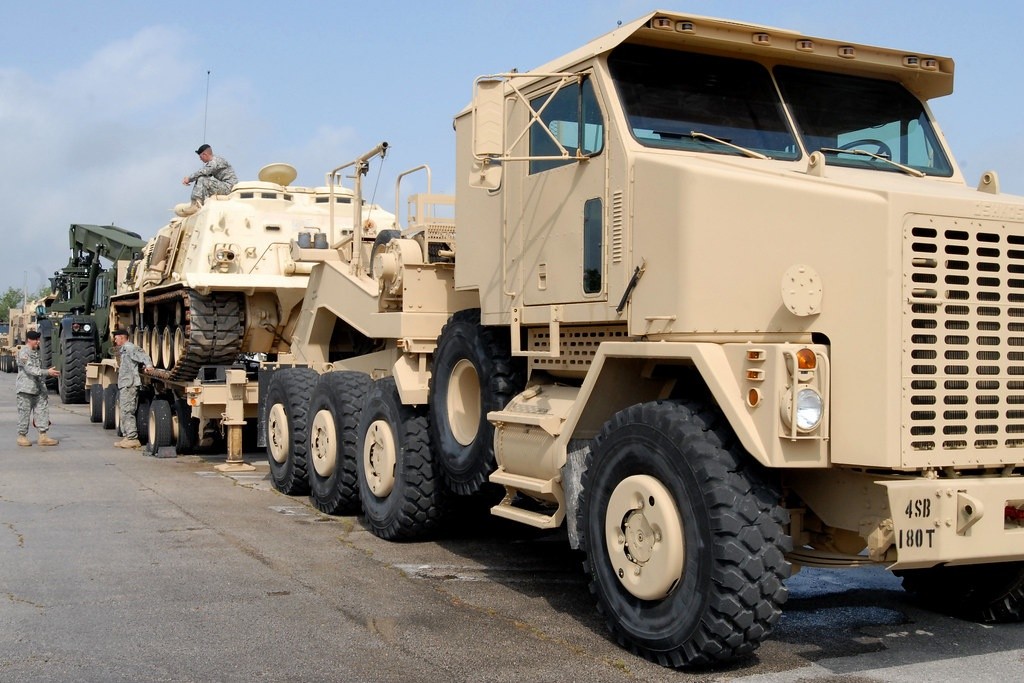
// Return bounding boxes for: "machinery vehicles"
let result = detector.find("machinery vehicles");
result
[1,162,407,455]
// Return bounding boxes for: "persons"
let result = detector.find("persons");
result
[181,144,238,215]
[15,330,61,447]
[111,329,154,449]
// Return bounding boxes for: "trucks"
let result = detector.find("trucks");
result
[260,11,1024,671]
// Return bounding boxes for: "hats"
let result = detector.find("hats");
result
[195,144,210,155]
[26,331,41,339]
[112,330,128,335]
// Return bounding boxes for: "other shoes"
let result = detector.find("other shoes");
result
[17,436,32,446]
[120,439,141,448]
[38,437,58,445]
[182,206,198,214]
[114,438,127,447]
